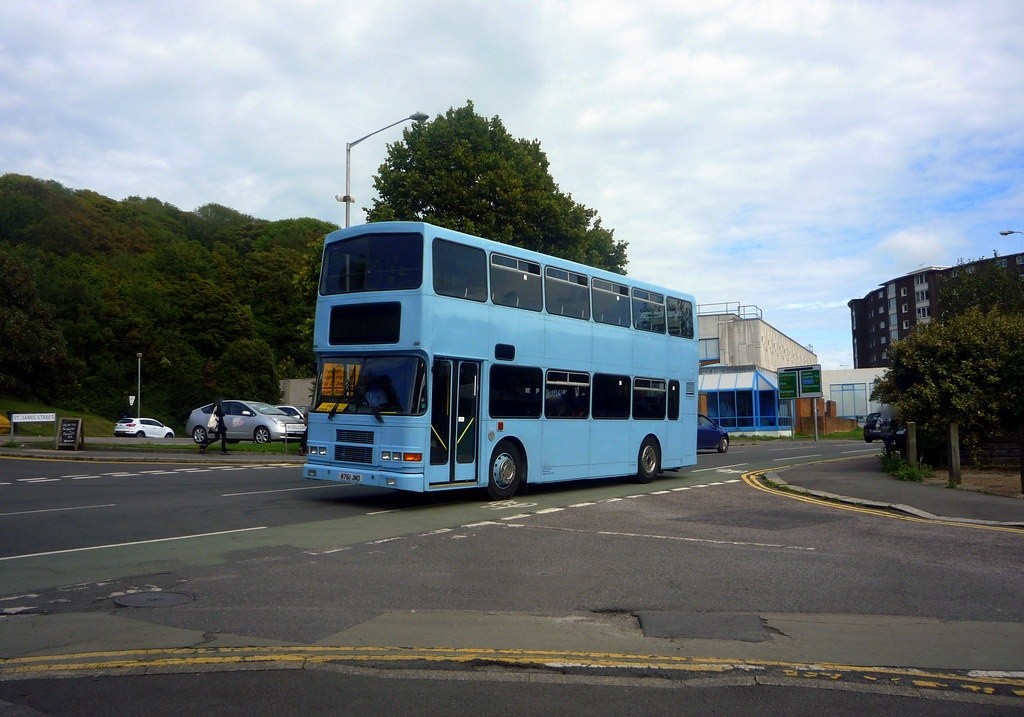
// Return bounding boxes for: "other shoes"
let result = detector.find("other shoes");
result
[220,451,231,455]
[199,445,205,454]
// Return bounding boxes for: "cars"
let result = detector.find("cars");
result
[698,413,729,455]
[186,401,308,445]
[114,418,175,439]
[273,406,312,421]
[863,413,895,443]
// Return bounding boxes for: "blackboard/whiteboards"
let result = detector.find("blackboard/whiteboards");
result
[55,417,84,451]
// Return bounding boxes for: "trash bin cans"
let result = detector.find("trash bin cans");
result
[0,415,11,433]
[6,411,19,434]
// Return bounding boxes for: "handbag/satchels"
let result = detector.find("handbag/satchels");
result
[207,413,219,432]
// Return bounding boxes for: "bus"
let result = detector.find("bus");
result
[302,221,699,499]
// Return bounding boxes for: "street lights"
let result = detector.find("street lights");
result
[136,353,142,418]
[342,114,432,395]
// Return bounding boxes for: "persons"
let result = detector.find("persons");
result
[200,397,227,455]
[355,375,387,409]
